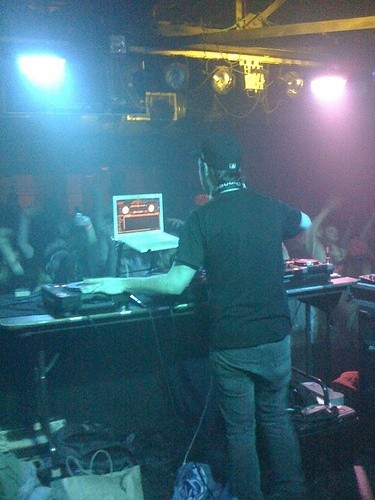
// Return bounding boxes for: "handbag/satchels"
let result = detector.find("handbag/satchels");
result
[50,450,144,500]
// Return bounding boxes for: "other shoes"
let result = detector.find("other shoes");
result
[13,275,32,298]
[38,268,55,285]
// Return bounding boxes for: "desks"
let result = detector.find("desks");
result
[0,273,358,471]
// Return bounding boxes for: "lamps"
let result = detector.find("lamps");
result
[207,66,236,95]
[149,60,189,89]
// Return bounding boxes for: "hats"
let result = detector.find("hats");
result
[190,132,241,170]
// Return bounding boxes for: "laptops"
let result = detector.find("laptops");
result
[111,192,181,252]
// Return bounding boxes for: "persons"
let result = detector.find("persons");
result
[0,198,375,337]
[80,136,312,500]
